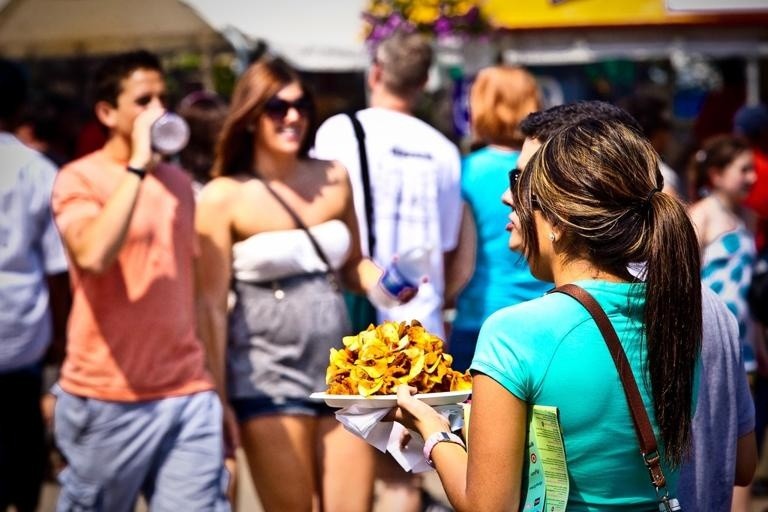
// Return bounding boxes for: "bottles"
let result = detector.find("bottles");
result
[149,114,190,153]
[368,243,435,310]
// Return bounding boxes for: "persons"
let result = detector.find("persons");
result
[0,33,766,510]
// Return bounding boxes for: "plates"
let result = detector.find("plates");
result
[308,388,473,408]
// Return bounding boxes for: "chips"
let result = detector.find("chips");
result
[326,318,473,397]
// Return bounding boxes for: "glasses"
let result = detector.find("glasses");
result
[508,168,520,192]
[263,95,310,120]
